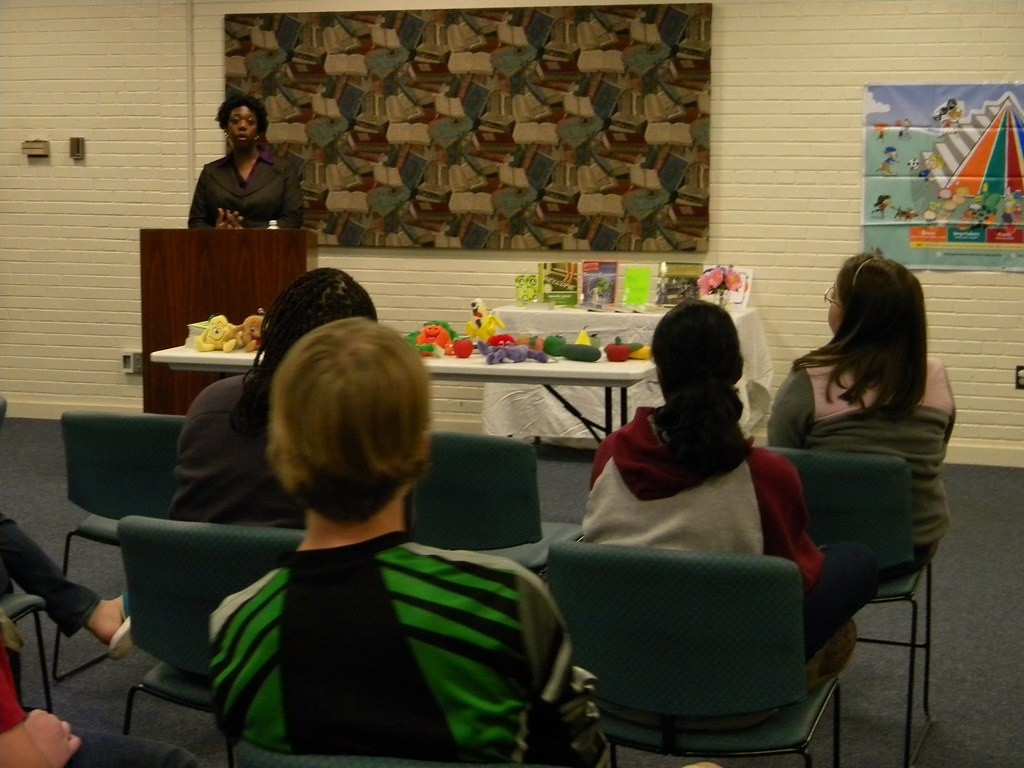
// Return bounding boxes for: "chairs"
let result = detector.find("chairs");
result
[50,410,188,683]
[412,432,586,580]
[0,593,53,714]
[547,539,859,768]
[232,739,559,768]
[117,515,307,768]
[756,442,932,768]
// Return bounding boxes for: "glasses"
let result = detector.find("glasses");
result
[824,285,845,310]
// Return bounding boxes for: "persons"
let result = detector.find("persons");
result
[1,615,200,768]
[165,261,613,766]
[579,297,877,737]
[766,251,958,585]
[186,94,303,230]
[1,395,138,660]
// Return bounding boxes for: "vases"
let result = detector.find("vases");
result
[716,290,729,308]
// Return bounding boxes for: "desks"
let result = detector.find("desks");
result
[493,302,759,448]
[150,343,660,445]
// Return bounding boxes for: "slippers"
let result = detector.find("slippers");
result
[107,593,143,662]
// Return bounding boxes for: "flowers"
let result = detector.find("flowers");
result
[696,262,743,297]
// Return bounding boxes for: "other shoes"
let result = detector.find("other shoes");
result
[804,617,858,691]
